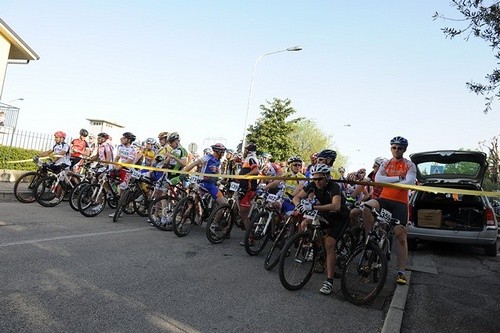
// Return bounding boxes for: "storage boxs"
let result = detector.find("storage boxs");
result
[418,209,441,228]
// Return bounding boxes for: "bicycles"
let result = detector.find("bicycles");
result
[341,202,406,305]
[14,153,365,290]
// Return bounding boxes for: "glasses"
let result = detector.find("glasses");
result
[373,164,378,167]
[391,147,403,150]
[314,177,323,181]
[55,137,58,138]
[293,164,301,167]
[319,158,326,161]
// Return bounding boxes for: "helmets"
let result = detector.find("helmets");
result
[203,143,272,161]
[146,131,181,147]
[287,157,303,165]
[310,136,409,181]
[54,128,136,145]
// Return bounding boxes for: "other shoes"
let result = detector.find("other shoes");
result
[93,205,167,226]
[240,239,255,246]
[320,281,333,294]
[49,197,59,204]
[396,272,407,284]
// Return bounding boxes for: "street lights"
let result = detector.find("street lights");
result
[241,46,302,155]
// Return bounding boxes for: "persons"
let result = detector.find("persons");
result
[33,128,337,246]
[363,137,417,282]
[337,158,388,225]
[292,163,351,294]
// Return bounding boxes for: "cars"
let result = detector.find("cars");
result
[406,150,498,256]
